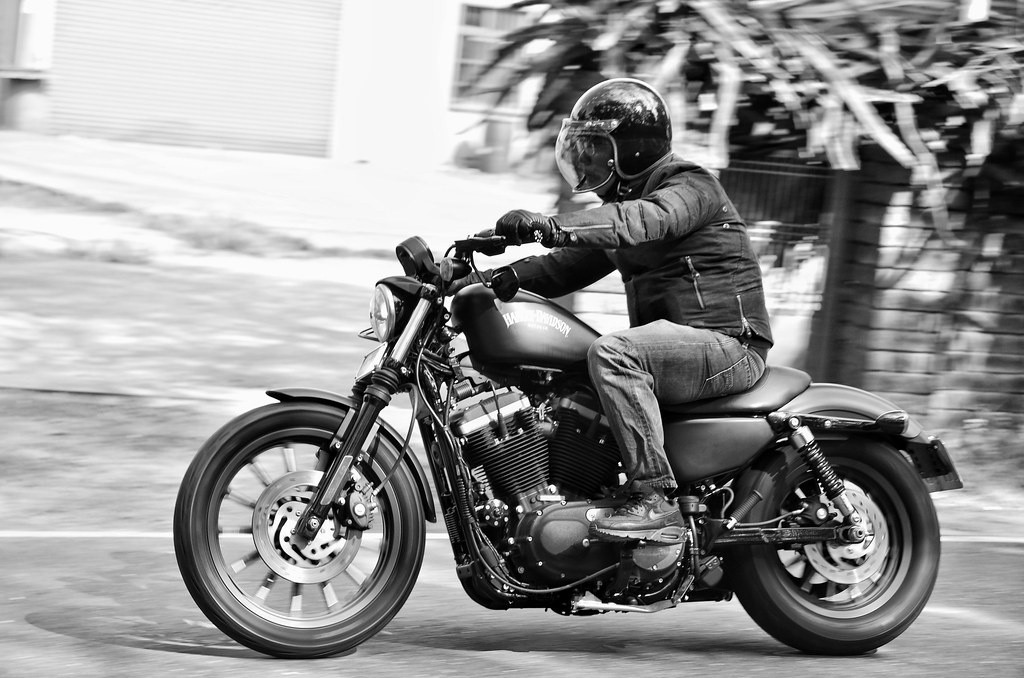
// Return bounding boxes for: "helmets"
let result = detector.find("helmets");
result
[568,76,676,181]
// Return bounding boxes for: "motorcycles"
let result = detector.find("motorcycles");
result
[172,224,963,658]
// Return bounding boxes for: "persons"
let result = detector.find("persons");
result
[435,77,778,546]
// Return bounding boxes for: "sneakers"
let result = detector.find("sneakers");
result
[588,483,688,545]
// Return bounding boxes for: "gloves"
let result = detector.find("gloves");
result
[446,268,495,296]
[495,209,552,248]
